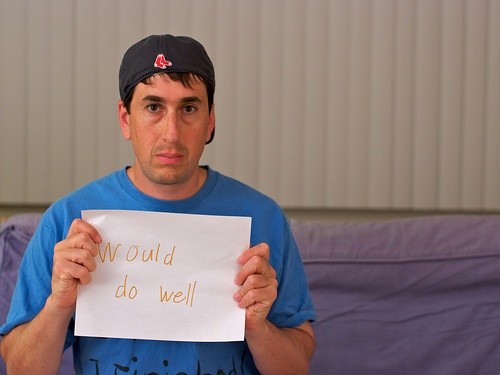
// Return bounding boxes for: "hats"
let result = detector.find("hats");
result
[118,34,215,145]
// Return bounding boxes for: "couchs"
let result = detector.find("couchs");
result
[0,212,499,375]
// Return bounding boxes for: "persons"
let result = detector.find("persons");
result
[1,33,317,375]
